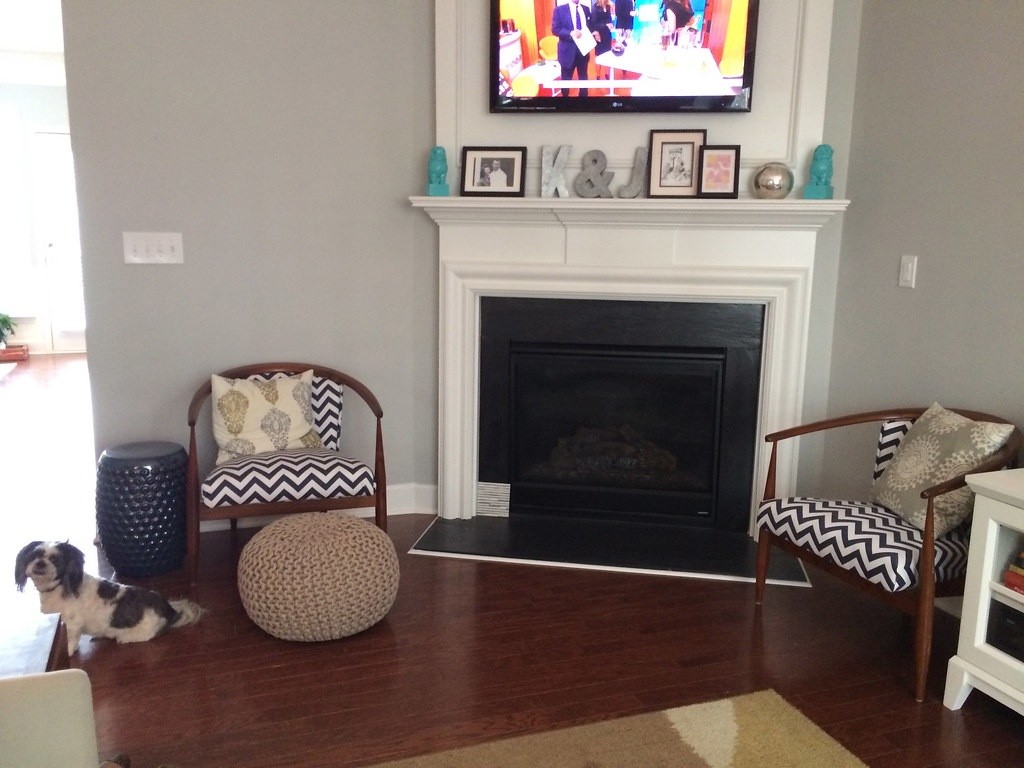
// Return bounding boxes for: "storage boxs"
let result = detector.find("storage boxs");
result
[1003,571,1024,594]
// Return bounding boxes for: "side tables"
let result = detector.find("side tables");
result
[942,466,1024,717]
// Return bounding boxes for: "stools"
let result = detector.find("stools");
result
[239,514,399,641]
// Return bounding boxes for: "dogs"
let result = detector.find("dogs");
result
[14,540,207,660]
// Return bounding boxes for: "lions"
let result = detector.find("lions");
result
[807,144,834,186]
[426,145,449,183]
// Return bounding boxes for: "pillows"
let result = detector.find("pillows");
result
[872,400,1016,540]
[210,368,326,468]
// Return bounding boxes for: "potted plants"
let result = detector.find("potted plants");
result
[0,312,29,362]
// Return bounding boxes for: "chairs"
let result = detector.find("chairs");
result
[753,408,1024,705]
[188,362,388,586]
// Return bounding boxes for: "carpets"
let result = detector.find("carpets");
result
[406,515,812,588]
[370,688,868,768]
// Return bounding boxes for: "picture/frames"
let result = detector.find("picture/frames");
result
[697,144,741,199]
[460,146,527,197]
[646,129,707,199]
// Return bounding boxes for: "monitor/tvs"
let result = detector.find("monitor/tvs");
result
[489,0,760,113]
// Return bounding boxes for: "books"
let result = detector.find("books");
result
[1003,545,1024,595]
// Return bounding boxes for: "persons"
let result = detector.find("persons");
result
[551,0,695,97]
[477,159,507,186]
